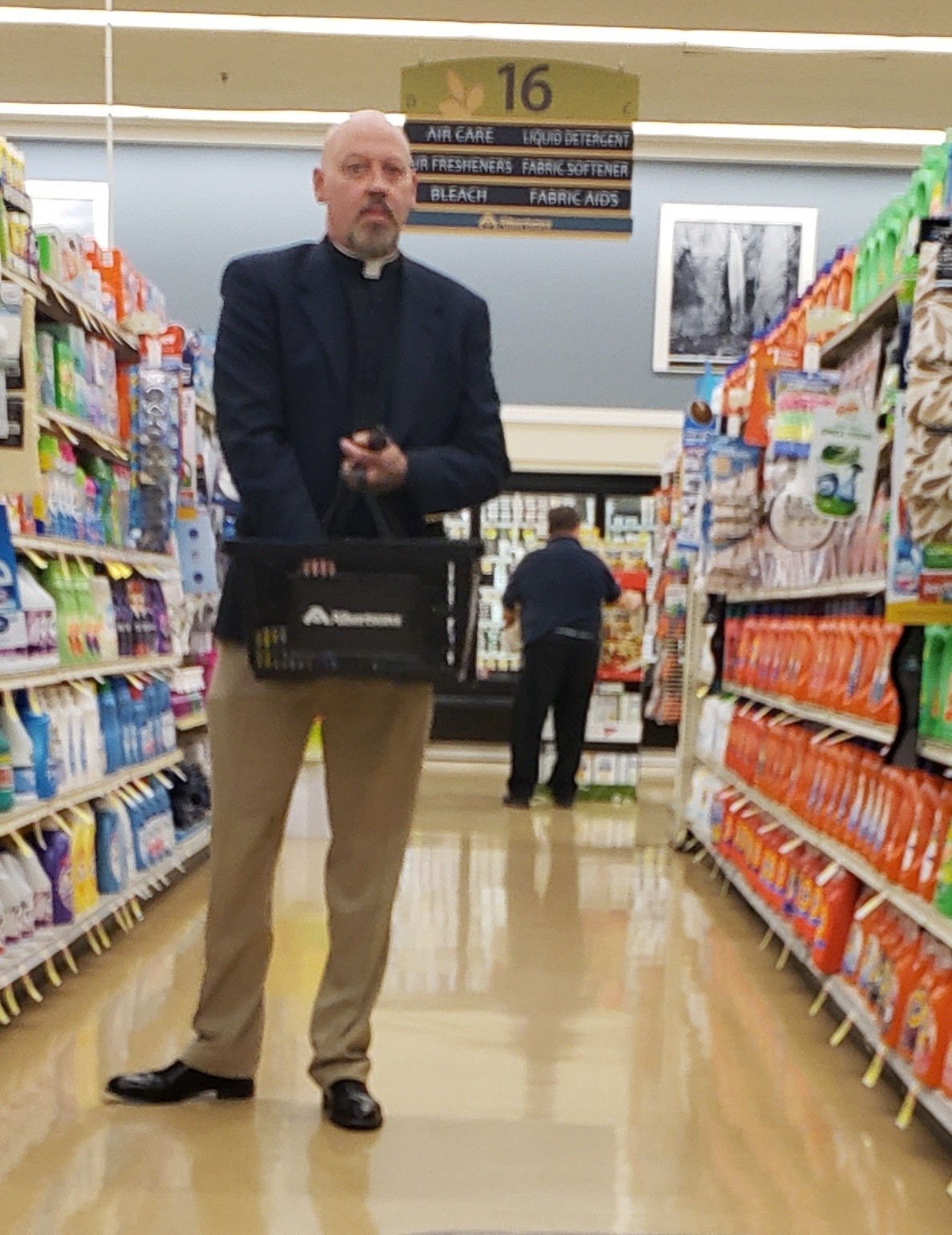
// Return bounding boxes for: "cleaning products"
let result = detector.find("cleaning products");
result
[690,142,949,1099]
[0,322,181,975]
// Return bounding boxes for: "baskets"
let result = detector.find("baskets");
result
[220,465,486,683]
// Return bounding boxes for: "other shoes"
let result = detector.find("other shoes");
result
[502,795,530,808]
[552,797,573,808]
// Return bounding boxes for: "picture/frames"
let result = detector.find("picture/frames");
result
[652,203,819,375]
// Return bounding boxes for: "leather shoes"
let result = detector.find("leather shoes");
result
[324,1078,383,1131]
[105,1059,255,1100]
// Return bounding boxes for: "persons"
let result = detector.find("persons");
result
[502,506,622,809]
[109,109,512,1131]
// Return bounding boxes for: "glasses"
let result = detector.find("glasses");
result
[367,422,388,451]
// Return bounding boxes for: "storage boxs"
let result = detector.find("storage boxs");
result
[540,682,643,799]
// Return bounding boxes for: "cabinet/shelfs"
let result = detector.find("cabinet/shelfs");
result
[675,266,952,1161]
[1,263,220,1019]
